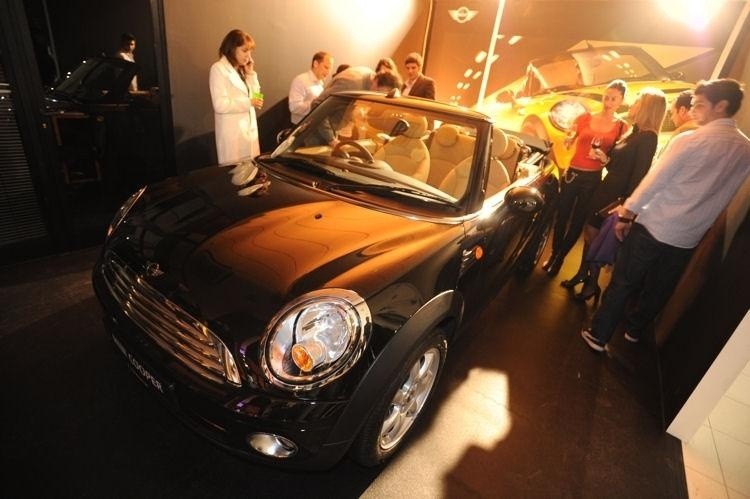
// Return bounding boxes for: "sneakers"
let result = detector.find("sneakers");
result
[581,328,605,352]
[625,332,639,343]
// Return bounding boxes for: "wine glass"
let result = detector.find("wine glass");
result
[585,133,603,160]
[563,122,580,147]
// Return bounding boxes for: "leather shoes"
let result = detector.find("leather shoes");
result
[542,253,557,270]
[548,256,563,275]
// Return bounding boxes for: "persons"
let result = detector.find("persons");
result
[286,49,435,149]
[208,29,265,161]
[232,163,275,201]
[543,81,750,353]
[119,31,139,89]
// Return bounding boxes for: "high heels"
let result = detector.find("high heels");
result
[570,286,601,302]
[561,275,589,288]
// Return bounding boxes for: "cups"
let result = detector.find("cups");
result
[251,93,264,110]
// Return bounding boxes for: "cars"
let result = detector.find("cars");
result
[92,92,562,476]
[498,45,696,171]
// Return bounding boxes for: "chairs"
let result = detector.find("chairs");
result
[370,114,519,201]
